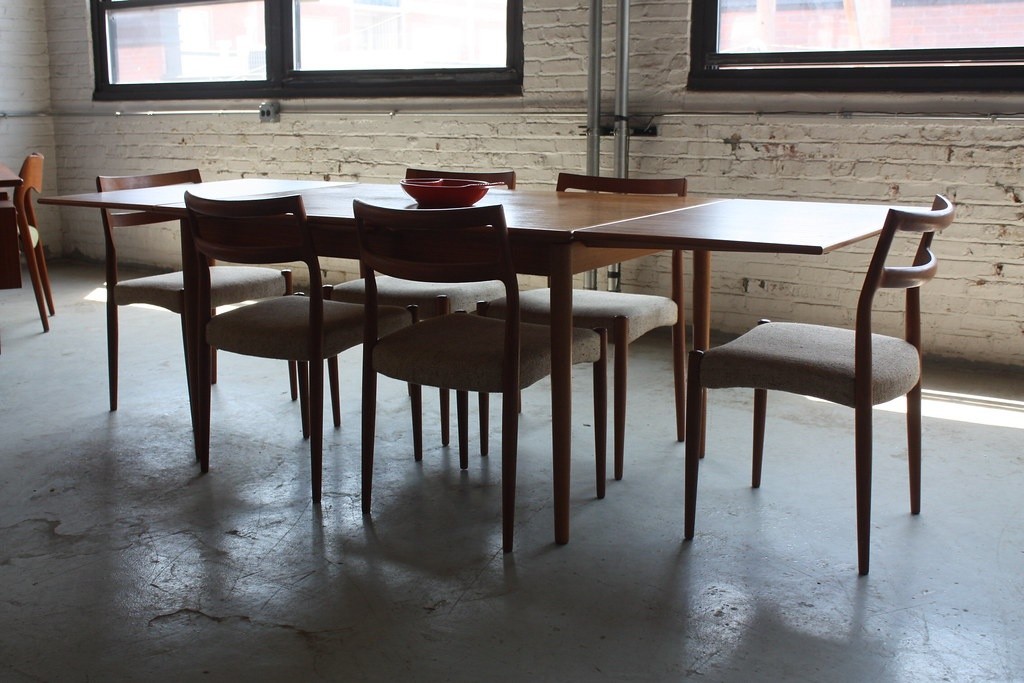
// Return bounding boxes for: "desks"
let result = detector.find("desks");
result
[37,179,932,544]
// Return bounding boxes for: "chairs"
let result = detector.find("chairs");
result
[684,193,953,576]
[94,170,298,461]
[183,169,684,552]
[0,152,55,331]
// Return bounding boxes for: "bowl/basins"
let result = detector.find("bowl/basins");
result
[400,177,490,209]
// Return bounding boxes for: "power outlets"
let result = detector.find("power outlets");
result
[258,102,279,123]
